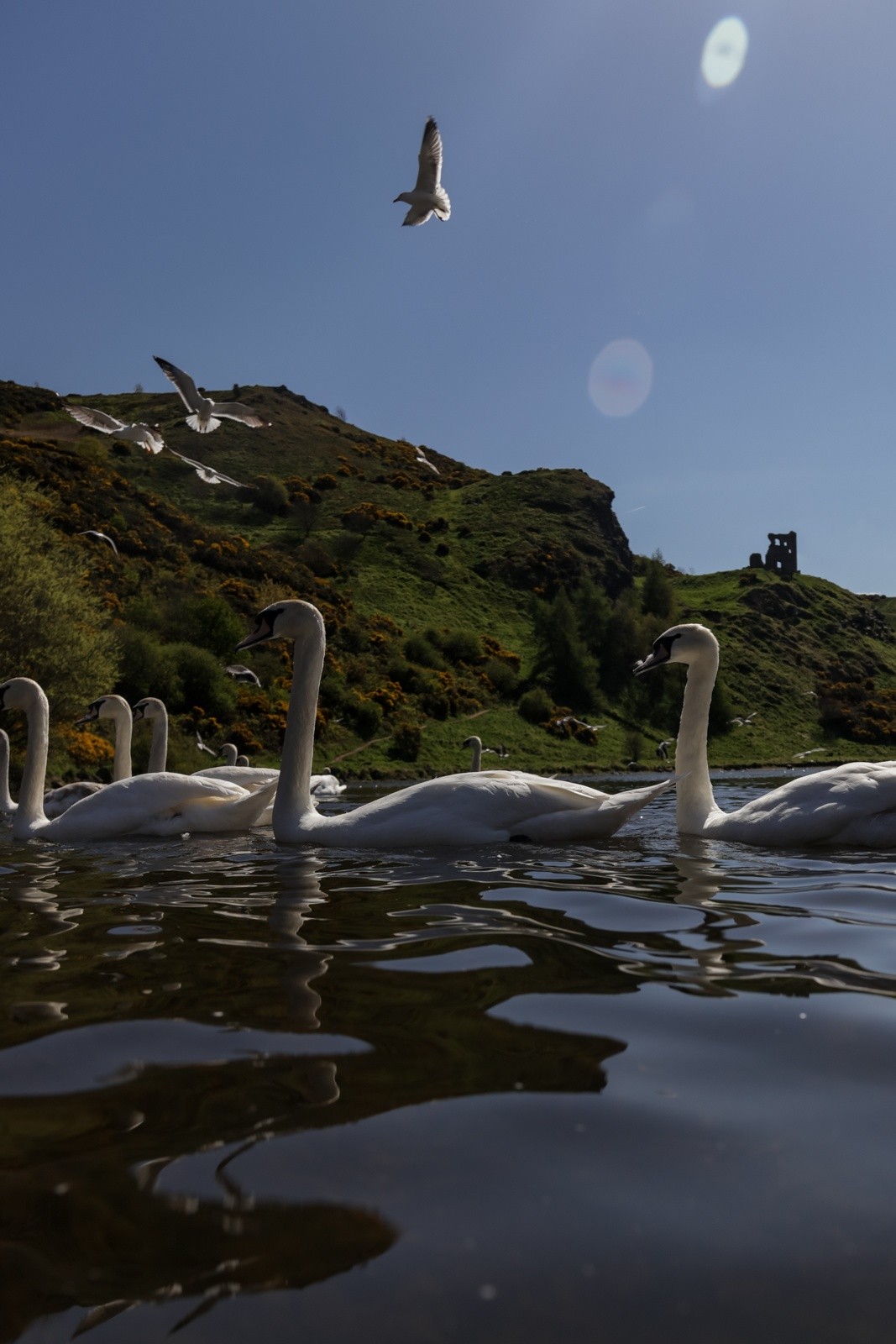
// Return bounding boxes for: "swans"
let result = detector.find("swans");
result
[0,598,896,851]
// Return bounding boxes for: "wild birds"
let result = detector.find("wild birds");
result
[391,116,454,227]
[153,353,274,435]
[164,447,252,489]
[62,403,165,456]
